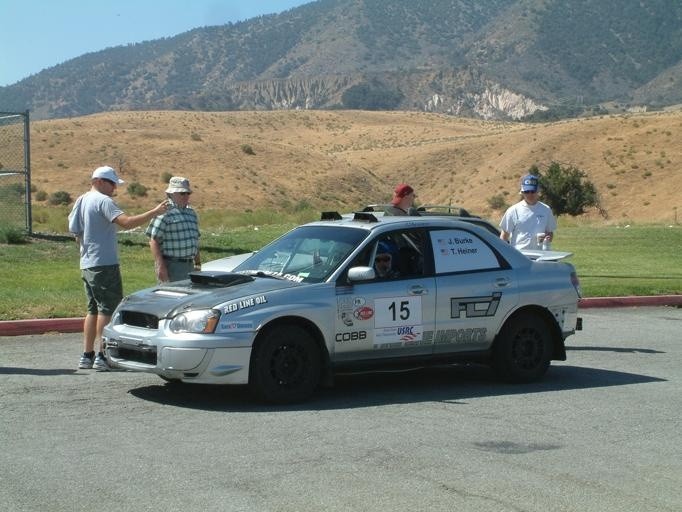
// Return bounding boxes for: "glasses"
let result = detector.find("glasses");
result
[374,256,391,263]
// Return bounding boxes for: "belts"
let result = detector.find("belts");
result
[162,254,195,263]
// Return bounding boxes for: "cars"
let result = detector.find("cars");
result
[100,209,583,405]
[200,201,572,279]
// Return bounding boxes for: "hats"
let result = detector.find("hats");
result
[518,173,539,192]
[369,240,394,255]
[388,184,414,207]
[91,164,125,186]
[163,176,194,195]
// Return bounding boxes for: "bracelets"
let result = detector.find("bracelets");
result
[195,262,201,266]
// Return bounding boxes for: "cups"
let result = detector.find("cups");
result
[536,233,546,247]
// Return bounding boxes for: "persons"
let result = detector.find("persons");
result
[145,176,202,287]
[67,165,173,372]
[383,185,414,216]
[371,243,400,279]
[498,175,555,251]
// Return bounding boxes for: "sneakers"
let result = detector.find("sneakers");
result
[78,352,96,368]
[94,351,129,372]
